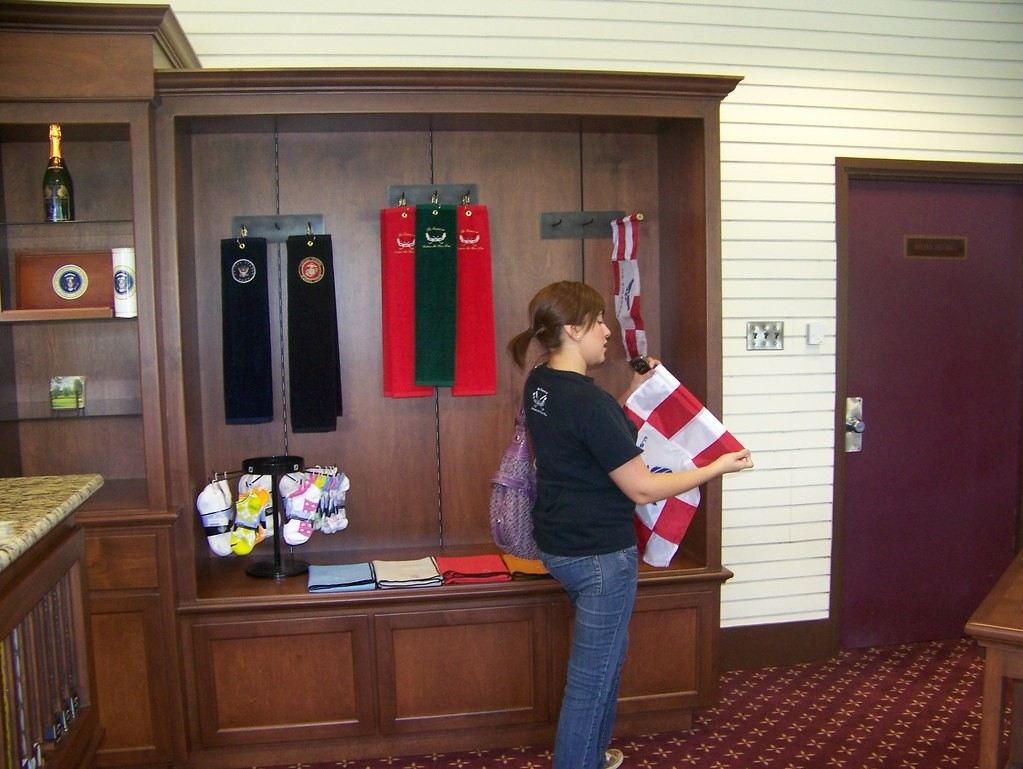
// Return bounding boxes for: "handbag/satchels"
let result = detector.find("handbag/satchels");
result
[489,361,544,559]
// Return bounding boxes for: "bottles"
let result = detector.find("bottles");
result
[43,122,76,223]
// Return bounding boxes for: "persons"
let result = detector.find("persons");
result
[507,281,754,769]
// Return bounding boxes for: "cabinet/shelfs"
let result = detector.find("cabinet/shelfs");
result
[1,0,745,769]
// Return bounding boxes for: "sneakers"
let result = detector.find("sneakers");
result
[605,749,624,769]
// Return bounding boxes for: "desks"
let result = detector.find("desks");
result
[963,550,1023,769]
